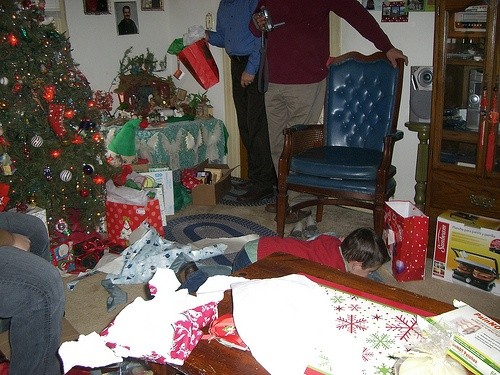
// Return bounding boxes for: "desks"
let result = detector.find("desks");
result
[96,115,229,210]
[403,121,431,213]
[144,250,500,375]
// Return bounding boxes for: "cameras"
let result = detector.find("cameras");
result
[259,8,285,32]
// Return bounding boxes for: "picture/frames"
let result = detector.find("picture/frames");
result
[114,1,140,36]
[141,0,165,11]
[83,0,112,16]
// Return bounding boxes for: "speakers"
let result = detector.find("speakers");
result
[409,66,433,123]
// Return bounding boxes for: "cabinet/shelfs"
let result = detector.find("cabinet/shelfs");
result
[423,0,500,260]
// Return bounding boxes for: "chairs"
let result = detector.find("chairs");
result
[276,50,406,262]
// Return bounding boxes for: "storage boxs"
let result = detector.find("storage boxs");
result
[70,236,106,270]
[6,203,50,239]
[191,157,242,206]
[432,209,500,296]
[49,229,76,275]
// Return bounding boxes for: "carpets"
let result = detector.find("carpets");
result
[216,175,276,207]
[161,214,276,246]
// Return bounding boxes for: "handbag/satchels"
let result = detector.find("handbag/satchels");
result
[177,37,220,89]
[101,159,176,247]
[382,198,428,281]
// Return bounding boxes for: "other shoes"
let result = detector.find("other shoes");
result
[238,187,274,203]
[265,202,289,212]
[177,260,198,284]
[234,178,256,189]
[273,205,311,223]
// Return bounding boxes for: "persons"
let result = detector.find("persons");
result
[248,0,409,226]
[200,0,280,202]
[176,228,391,296]
[118,6,138,35]
[0,211,67,375]
